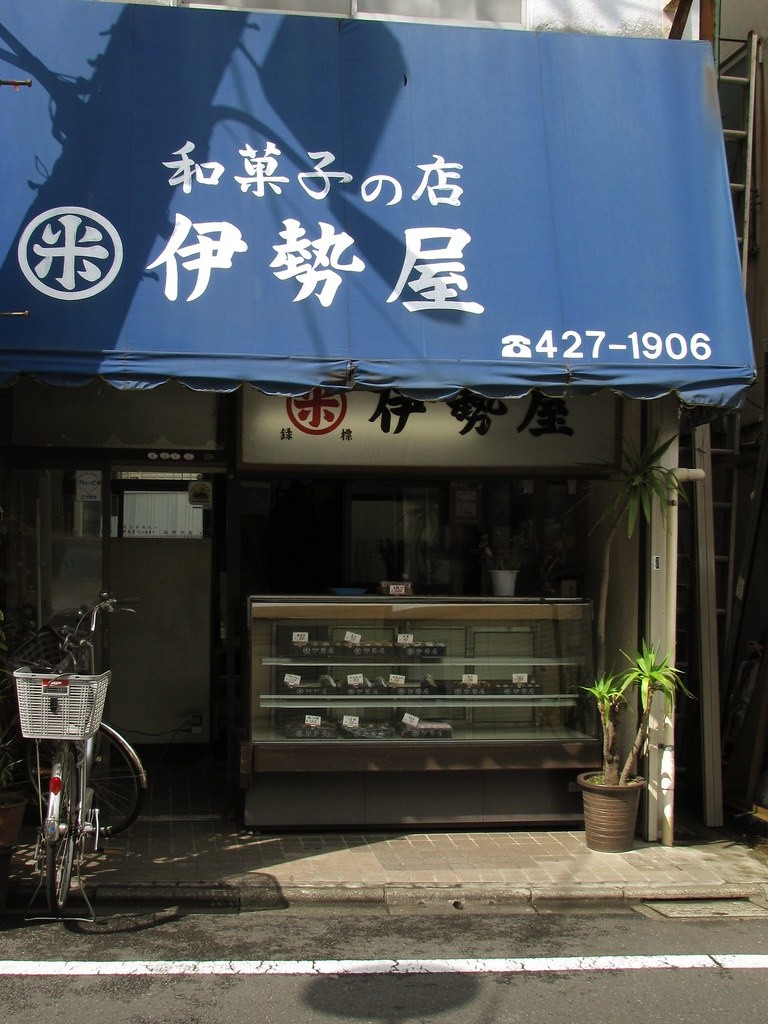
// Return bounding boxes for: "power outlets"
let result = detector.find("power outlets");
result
[189,712,205,735]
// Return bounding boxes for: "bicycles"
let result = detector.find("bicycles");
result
[12,589,148,927]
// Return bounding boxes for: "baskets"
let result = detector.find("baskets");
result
[13,666,111,740]
[37,630,63,663]
[5,626,40,663]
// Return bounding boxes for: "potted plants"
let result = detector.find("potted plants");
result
[480,534,521,596]
[0,609,29,846]
[578,639,698,853]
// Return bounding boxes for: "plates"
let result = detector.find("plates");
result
[330,588,368,595]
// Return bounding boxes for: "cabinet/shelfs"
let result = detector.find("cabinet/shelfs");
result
[240,595,594,826]
